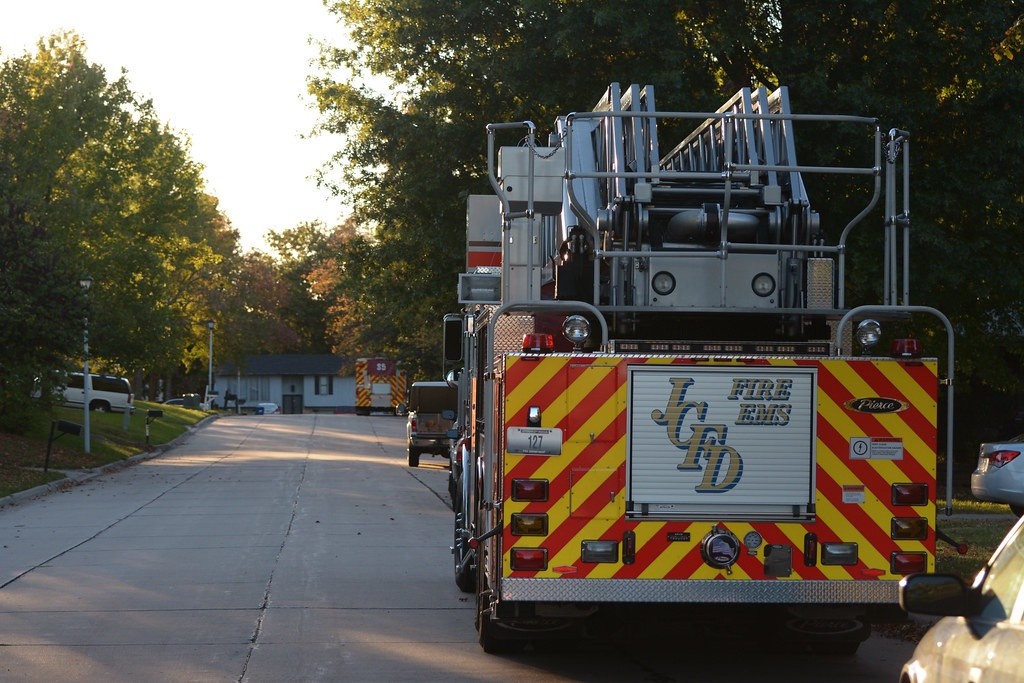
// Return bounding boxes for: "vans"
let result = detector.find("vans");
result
[30,370,136,414]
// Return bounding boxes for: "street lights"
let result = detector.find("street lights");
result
[207,320,215,393]
[78,274,93,455]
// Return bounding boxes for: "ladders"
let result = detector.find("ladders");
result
[660,86,802,206]
[588,80,660,200]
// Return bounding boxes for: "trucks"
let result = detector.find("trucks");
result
[354,356,408,417]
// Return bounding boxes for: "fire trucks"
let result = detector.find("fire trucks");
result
[442,83,956,657]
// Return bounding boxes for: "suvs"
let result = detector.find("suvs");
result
[396,381,460,467]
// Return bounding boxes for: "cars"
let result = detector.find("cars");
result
[163,398,210,414]
[971,433,1024,518]
[898,516,1023,683]
